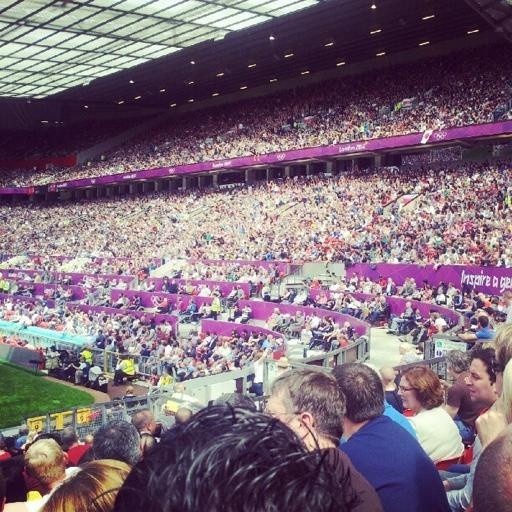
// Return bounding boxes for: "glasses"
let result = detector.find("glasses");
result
[398,385,414,392]
